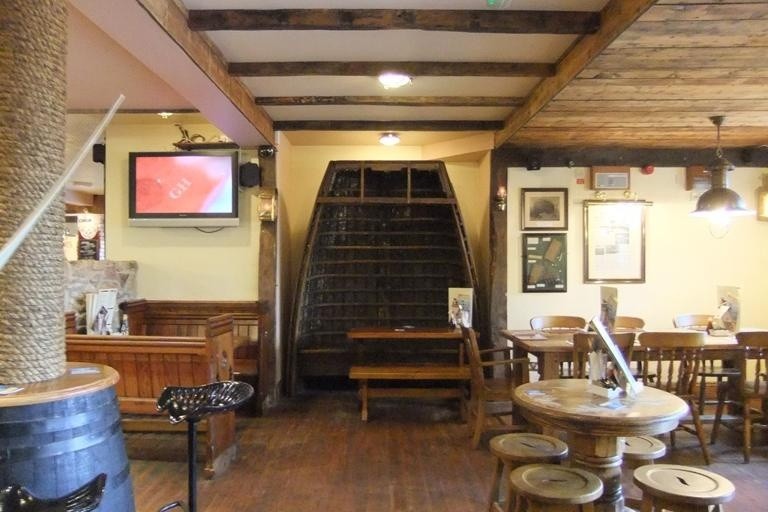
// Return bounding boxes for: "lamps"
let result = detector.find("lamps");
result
[378,72,411,91]
[379,130,401,151]
[691,117,758,230]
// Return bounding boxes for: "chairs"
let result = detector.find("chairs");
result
[674,314,742,423]
[590,316,643,334]
[571,331,637,386]
[461,325,532,450]
[640,331,709,467]
[532,314,584,338]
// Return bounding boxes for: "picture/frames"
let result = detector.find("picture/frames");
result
[582,200,650,286]
[522,188,571,232]
[522,231,568,295]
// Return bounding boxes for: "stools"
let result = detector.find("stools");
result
[622,435,667,512]
[509,462,604,512]
[154,381,254,512]
[632,463,736,512]
[484,433,569,512]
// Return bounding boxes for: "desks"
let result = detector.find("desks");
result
[347,325,483,367]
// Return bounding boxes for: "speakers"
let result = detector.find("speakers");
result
[92,143,104,163]
[239,161,259,187]
[526,148,543,171]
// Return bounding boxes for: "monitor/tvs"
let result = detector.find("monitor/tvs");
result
[127,151,240,227]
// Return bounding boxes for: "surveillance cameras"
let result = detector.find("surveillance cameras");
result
[258,145,273,158]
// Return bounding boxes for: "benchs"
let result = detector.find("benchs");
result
[349,370,477,424]
